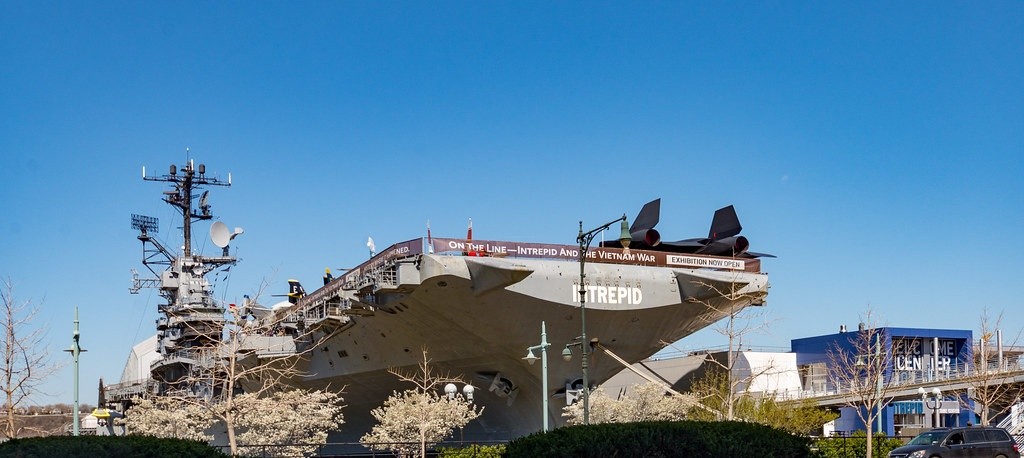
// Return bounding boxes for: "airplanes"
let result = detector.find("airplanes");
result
[599,197,779,260]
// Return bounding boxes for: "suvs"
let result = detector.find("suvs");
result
[887,424,1022,458]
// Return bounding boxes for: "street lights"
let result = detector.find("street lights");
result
[563,212,633,423]
[855,334,887,432]
[444,383,474,443]
[521,321,551,432]
[917,387,943,427]
[63,306,89,433]
